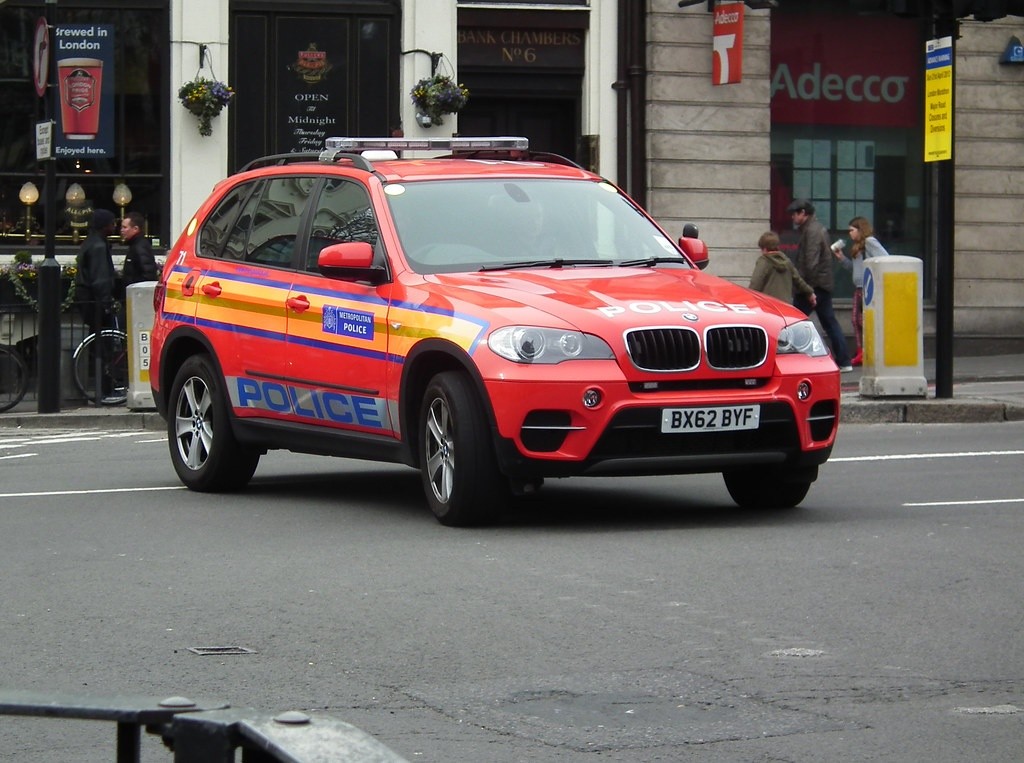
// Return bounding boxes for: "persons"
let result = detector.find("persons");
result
[749,232,817,307]
[788,198,853,372]
[120,214,158,355]
[76,209,124,401]
[834,216,889,365]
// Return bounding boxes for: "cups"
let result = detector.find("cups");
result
[830,238,846,251]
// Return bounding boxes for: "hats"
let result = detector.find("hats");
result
[786,199,812,211]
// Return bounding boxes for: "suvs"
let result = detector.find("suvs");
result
[148,135,842,527]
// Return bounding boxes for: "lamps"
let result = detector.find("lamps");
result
[113,183,132,223]
[19,182,39,235]
[65,183,86,235]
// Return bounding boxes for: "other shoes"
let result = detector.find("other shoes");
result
[838,364,853,373]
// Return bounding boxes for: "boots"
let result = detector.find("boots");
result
[851,348,863,366]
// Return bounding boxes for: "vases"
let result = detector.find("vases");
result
[0,279,127,305]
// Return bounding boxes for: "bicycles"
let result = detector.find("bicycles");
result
[0,343,31,415]
[70,295,129,408]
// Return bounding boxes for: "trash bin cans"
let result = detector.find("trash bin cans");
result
[859,254,928,397]
[125,281,159,408]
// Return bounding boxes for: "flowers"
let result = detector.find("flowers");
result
[0,251,166,313]
[409,73,471,126]
[177,77,235,138]
